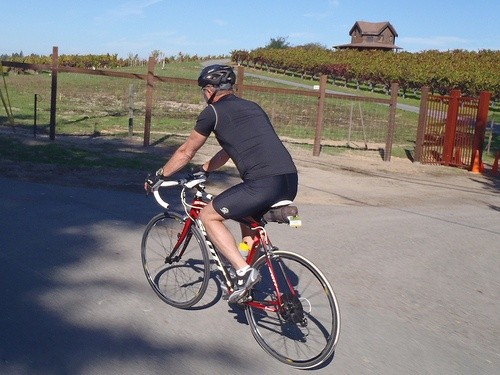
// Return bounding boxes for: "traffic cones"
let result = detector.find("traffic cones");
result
[453,146,463,163]
[468,149,481,175]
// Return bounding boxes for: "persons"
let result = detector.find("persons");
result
[144,65,298,303]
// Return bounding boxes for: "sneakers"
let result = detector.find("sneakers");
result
[228,267,261,303]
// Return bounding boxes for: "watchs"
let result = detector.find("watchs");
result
[157,168,168,180]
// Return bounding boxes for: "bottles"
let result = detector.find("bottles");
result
[230,241,249,275]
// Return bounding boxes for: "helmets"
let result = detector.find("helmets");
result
[197,64,236,86]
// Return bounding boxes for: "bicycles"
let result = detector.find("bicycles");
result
[141,168,342,369]
